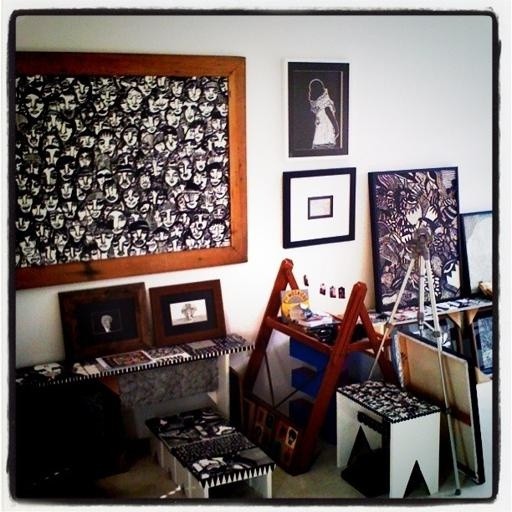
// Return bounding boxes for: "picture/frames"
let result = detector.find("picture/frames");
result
[14,50,248,290]
[288,62,349,158]
[149,279,227,347]
[57,282,153,363]
[367,166,464,313]
[282,167,356,249]
[460,210,493,298]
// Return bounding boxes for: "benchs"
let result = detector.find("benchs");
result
[17,333,256,448]
[145,407,275,498]
[330,297,493,388]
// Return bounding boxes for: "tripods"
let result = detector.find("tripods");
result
[366,229,462,497]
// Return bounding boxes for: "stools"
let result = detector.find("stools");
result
[336,376,442,499]
